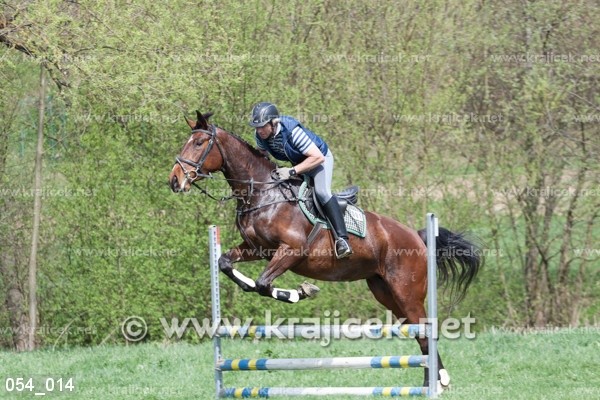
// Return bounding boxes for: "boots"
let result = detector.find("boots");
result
[322,195,353,258]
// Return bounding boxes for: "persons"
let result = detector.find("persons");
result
[248,102,353,257]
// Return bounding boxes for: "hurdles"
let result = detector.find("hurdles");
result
[213,324,438,400]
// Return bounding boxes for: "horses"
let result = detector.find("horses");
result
[167,109,487,394]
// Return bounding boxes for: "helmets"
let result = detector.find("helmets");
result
[248,102,278,127]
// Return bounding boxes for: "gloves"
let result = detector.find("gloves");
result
[277,165,297,181]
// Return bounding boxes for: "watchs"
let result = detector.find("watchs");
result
[289,168,296,176]
